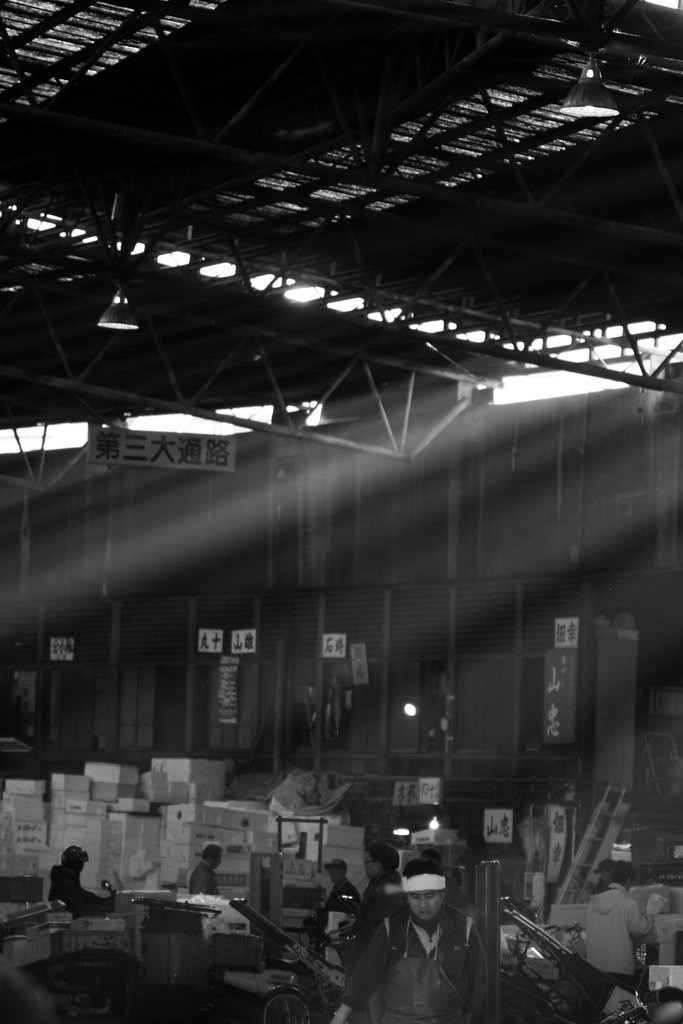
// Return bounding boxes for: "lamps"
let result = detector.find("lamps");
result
[559,46,620,119]
[95,279,141,333]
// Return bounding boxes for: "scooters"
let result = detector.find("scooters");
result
[599,942,683,1024]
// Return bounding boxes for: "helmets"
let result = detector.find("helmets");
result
[61,845,89,872]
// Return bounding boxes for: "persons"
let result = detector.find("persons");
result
[319,860,361,942]
[421,850,465,908]
[48,845,114,918]
[189,844,223,896]
[586,860,653,984]
[343,842,406,1024]
[331,857,490,1024]
[592,859,615,895]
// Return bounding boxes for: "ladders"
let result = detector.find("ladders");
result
[546,800,631,924]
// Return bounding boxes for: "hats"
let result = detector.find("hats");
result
[324,859,347,871]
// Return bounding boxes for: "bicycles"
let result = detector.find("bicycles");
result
[256,927,356,1024]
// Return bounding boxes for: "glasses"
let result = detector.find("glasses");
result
[364,860,379,864]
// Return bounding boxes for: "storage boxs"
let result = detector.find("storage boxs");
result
[0,753,458,968]
[550,881,683,966]
